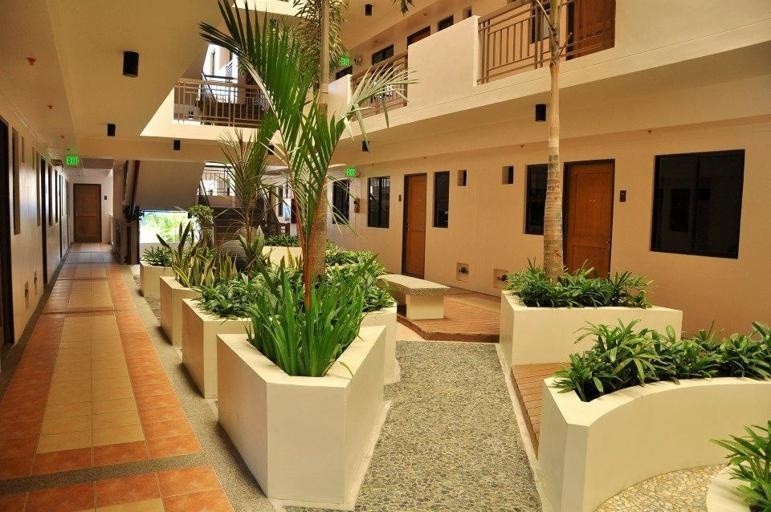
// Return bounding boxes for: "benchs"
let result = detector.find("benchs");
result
[378,272,452,322]
